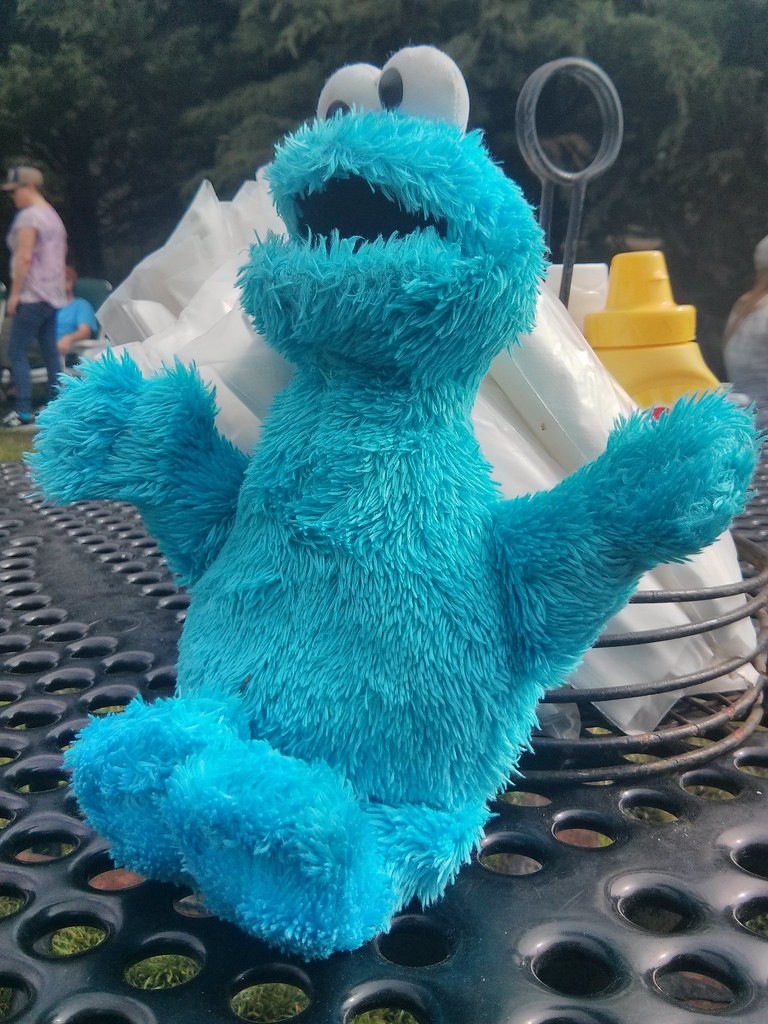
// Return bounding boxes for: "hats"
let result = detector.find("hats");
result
[0,167,44,190]
[65,267,76,281]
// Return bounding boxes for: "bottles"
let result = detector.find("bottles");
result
[583,250,722,427]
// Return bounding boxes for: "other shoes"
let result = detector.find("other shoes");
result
[32,398,57,415]
[1,411,35,427]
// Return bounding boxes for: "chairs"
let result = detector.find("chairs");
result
[0,277,112,395]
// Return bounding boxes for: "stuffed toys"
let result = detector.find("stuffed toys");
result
[21,44,768,967]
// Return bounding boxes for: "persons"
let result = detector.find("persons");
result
[0,167,68,430]
[718,235,768,426]
[55,267,99,366]
